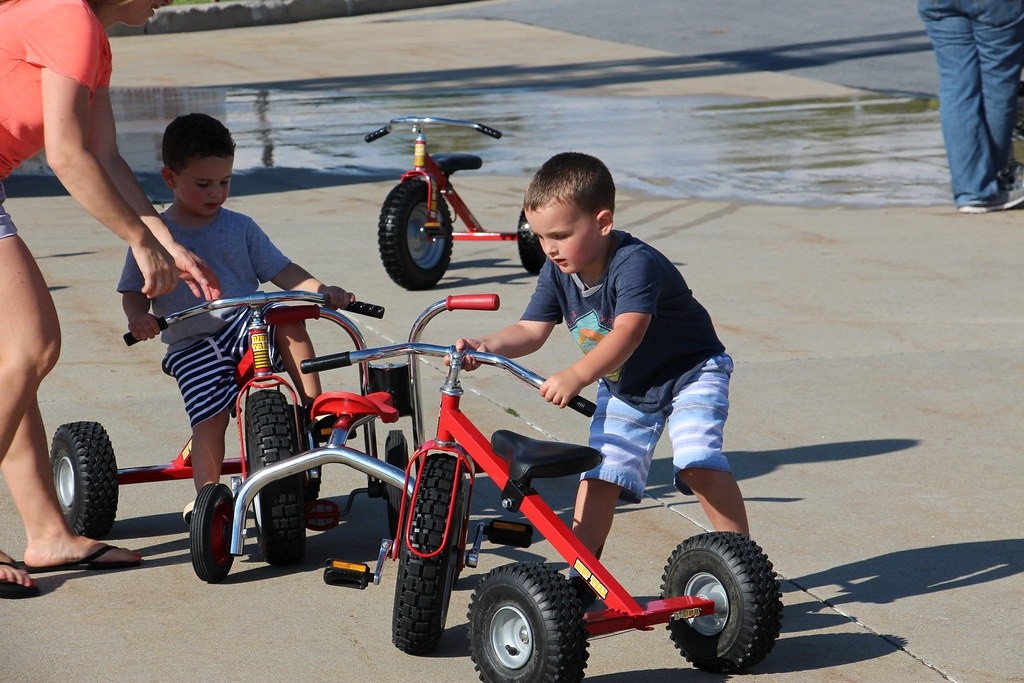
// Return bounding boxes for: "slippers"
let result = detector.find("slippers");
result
[0,561,40,599]
[27,545,144,573]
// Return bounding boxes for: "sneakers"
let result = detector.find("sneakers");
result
[183,501,194,525]
[311,414,333,439]
[1000,162,1024,189]
[958,184,1024,213]
[569,576,600,613]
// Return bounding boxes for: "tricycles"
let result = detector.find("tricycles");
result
[186,295,502,584]
[292,340,784,683]
[47,286,387,564]
[358,115,556,292]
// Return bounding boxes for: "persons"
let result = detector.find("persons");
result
[915,0,1024,215]
[0,0,176,600]
[443,150,750,614]
[115,112,357,529]
